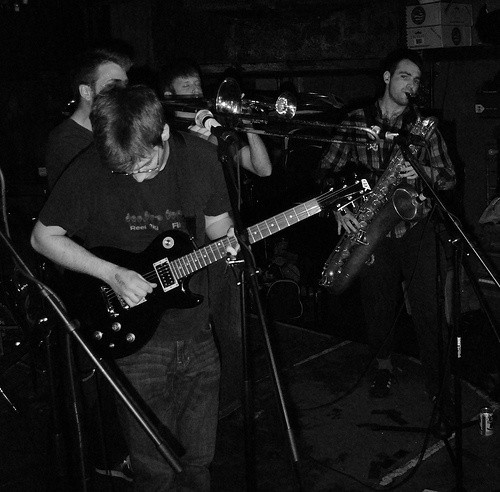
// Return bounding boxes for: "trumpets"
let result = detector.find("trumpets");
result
[194,90,297,122]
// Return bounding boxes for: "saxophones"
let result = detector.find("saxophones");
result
[317,92,438,299]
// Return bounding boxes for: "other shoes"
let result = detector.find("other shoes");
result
[96,457,133,481]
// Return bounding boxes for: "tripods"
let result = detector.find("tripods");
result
[372,146,500,470]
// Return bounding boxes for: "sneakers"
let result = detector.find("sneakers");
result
[369,369,394,397]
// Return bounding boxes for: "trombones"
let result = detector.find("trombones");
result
[166,77,378,147]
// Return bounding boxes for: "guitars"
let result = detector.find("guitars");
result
[39,180,371,360]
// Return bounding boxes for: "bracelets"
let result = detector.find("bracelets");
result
[227,224,235,233]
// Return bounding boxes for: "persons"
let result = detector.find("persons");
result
[43,49,135,483]
[29,82,242,492]
[158,57,273,467]
[319,47,463,421]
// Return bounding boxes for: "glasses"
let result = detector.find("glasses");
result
[112,147,161,176]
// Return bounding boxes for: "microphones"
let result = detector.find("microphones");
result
[195,108,240,148]
[367,125,427,145]
